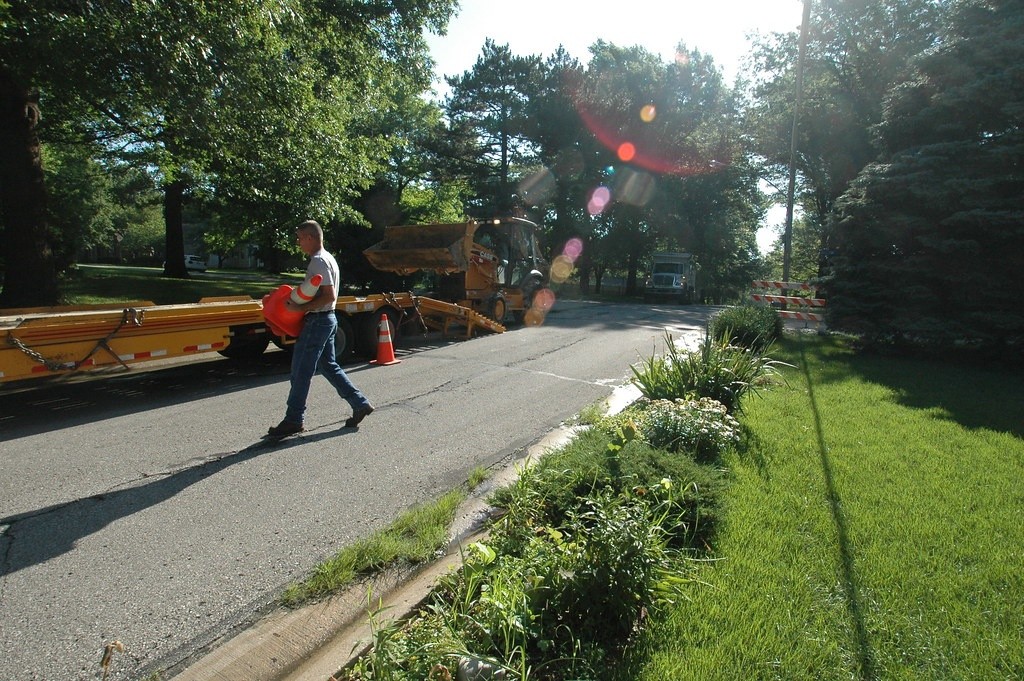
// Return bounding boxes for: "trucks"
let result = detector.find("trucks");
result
[645,253,697,306]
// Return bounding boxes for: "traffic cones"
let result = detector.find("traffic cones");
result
[370,314,400,365]
[262,274,323,337]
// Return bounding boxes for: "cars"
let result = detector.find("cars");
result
[185,255,207,271]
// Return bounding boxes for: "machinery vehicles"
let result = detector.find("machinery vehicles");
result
[364,215,552,326]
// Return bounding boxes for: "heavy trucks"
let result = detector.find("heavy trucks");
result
[2,289,505,387]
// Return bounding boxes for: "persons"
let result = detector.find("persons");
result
[266,220,374,436]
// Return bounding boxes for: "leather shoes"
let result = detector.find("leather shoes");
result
[346,403,374,427]
[268,420,304,437]
